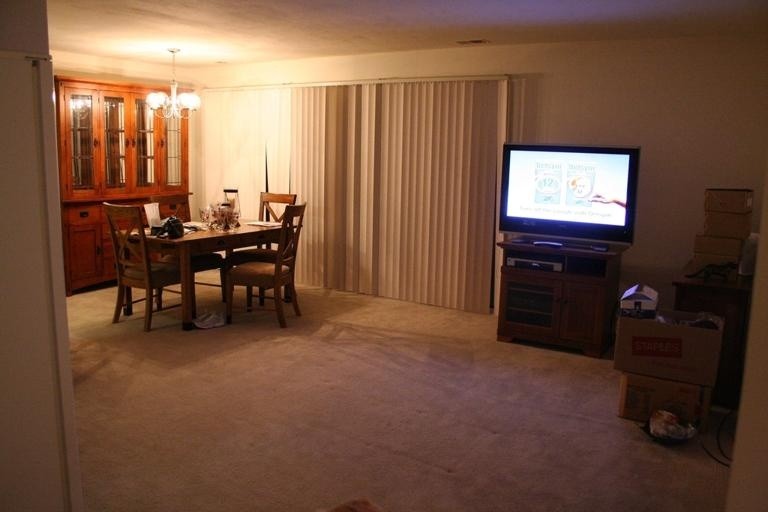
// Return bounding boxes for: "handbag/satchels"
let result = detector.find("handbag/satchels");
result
[156,217,183,239]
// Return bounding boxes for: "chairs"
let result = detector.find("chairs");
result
[103,190,307,332]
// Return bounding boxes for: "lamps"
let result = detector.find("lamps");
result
[146,41,200,119]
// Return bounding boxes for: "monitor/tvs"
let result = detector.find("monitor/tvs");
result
[499,143,641,252]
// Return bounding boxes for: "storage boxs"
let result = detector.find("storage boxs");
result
[692,188,755,267]
[610,297,726,428]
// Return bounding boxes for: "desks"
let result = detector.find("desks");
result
[668,263,753,410]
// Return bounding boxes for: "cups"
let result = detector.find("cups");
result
[197,207,240,234]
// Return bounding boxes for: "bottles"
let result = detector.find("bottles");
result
[218,188,240,226]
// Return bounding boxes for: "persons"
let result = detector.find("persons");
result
[587,193,626,211]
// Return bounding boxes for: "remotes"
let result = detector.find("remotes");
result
[534,240,563,248]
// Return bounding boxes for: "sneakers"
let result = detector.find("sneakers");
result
[192,310,224,328]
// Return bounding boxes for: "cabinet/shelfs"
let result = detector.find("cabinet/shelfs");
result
[64,87,101,199]
[101,90,156,199]
[495,235,631,360]
[63,205,105,293]
[156,95,192,194]
